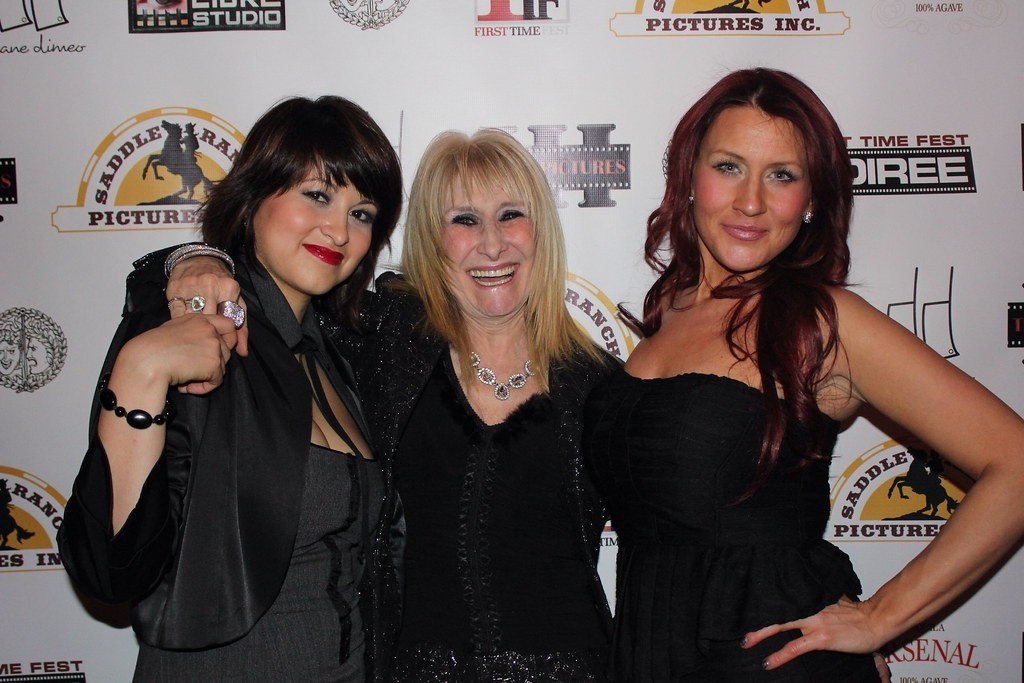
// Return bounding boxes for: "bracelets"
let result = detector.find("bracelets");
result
[164,243,236,280]
[97,371,171,429]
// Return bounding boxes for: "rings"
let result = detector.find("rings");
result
[184,296,205,311]
[222,300,245,328]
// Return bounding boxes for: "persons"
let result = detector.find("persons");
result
[56,94,406,683]
[161,128,625,683]
[587,67,1024,683]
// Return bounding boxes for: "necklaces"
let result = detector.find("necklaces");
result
[168,297,185,309]
[467,351,537,399]
